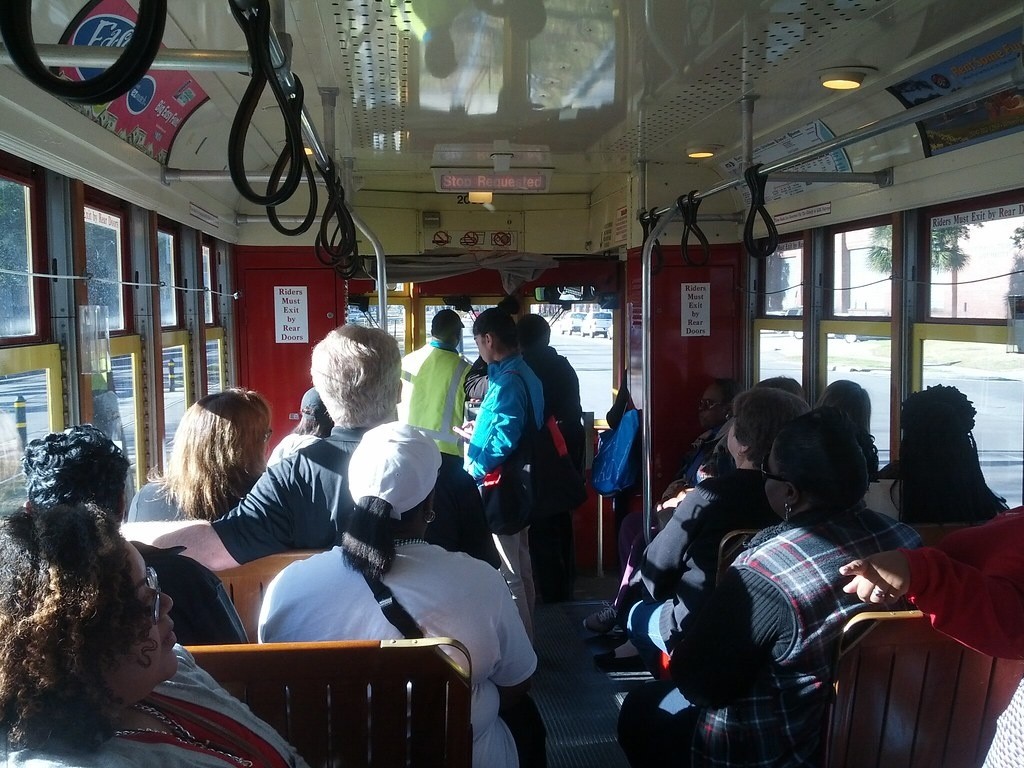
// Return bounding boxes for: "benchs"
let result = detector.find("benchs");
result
[183,550,472,768]
[717,529,1024,768]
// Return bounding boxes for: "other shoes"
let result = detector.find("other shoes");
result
[593,648,641,668]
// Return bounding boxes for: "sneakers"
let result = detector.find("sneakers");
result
[613,625,624,633]
[583,600,621,633]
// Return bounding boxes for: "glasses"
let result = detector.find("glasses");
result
[131,566,161,626]
[264,429,272,443]
[696,398,734,411]
[761,452,801,494]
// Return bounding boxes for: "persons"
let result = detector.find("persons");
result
[264,385,334,472]
[839,507,1023,660]
[118,325,502,571]
[617,407,929,768]
[879,385,1007,524]
[125,389,272,525]
[582,369,810,673]
[18,424,253,644]
[397,308,539,650]
[1,493,312,768]
[259,420,549,768]
[517,313,588,603]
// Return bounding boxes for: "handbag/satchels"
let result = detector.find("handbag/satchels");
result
[474,371,587,536]
[591,408,643,497]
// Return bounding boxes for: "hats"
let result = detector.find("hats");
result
[347,421,442,520]
[300,386,327,420]
[432,309,464,332]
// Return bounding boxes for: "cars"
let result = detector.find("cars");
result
[580,312,612,338]
[560,312,588,335]
